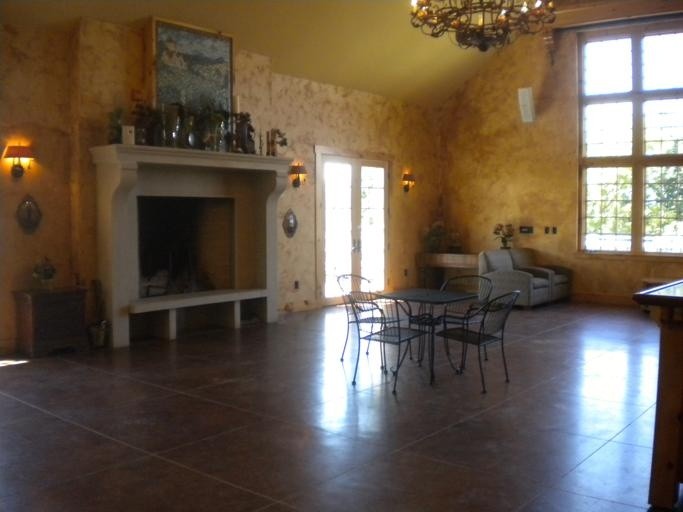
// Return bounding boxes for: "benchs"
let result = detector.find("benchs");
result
[129,287,265,341]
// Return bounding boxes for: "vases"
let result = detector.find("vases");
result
[42,279,53,290]
[104,98,288,158]
[500,238,508,247]
[427,239,440,253]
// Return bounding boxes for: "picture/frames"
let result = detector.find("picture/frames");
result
[142,18,239,115]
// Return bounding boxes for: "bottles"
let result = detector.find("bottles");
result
[153,104,254,153]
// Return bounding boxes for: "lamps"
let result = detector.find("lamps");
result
[409,0,563,53]
[3,145,41,177]
[400,174,416,193]
[288,162,308,187]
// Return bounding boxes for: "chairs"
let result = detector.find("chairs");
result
[346,290,435,393]
[407,272,494,374]
[426,289,521,393]
[336,273,412,369]
[479,249,551,310]
[509,248,575,303]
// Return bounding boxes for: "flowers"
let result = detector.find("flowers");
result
[30,255,58,278]
[492,222,513,241]
[419,220,446,242]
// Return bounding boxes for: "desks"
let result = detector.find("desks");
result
[415,253,478,289]
[372,286,480,390]
[632,279,682,511]
[11,285,88,359]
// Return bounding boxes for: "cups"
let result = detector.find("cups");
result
[136,128,145,144]
